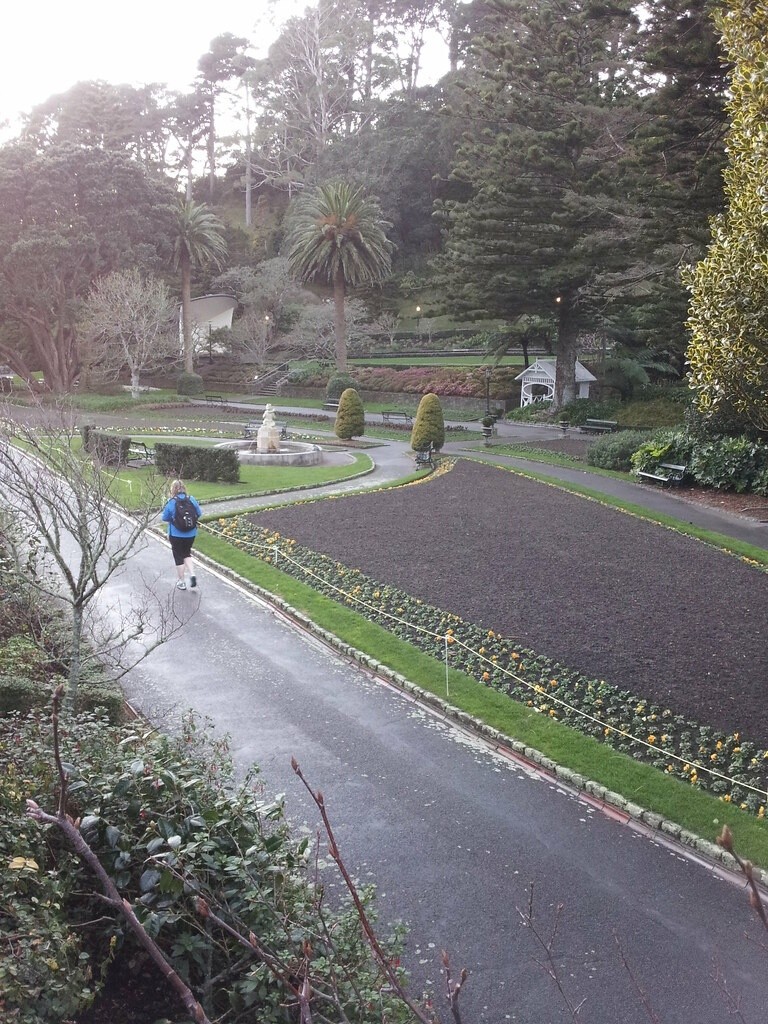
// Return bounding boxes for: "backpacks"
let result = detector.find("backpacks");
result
[170,495,198,531]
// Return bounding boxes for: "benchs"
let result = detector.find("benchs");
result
[205,395,229,406]
[578,419,617,437]
[638,462,688,490]
[382,411,414,424]
[322,398,341,410]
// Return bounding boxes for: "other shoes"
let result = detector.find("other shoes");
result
[190,574,197,587]
[176,580,186,590]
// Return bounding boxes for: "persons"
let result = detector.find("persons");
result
[162,480,201,590]
[262,403,276,427]
